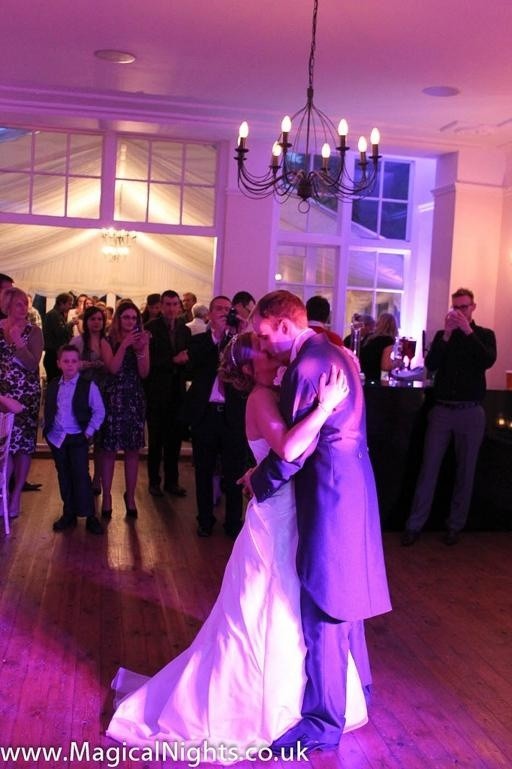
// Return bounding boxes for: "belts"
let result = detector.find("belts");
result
[439,402,476,409]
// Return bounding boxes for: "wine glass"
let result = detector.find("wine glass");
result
[394,336,417,372]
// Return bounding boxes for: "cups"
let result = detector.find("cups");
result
[505,370,511,390]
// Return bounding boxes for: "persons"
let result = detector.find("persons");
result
[105,331,369,766]
[1,274,405,540]
[236,289,394,757]
[401,289,498,546]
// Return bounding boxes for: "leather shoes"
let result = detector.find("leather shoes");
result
[445,528,456,545]
[402,531,419,545]
[8,478,243,539]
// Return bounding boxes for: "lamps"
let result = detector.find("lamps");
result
[96,144,136,265]
[231,0,385,216]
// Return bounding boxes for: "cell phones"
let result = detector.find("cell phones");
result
[133,325,141,340]
[449,311,457,319]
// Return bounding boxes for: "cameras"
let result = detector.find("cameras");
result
[222,308,242,327]
